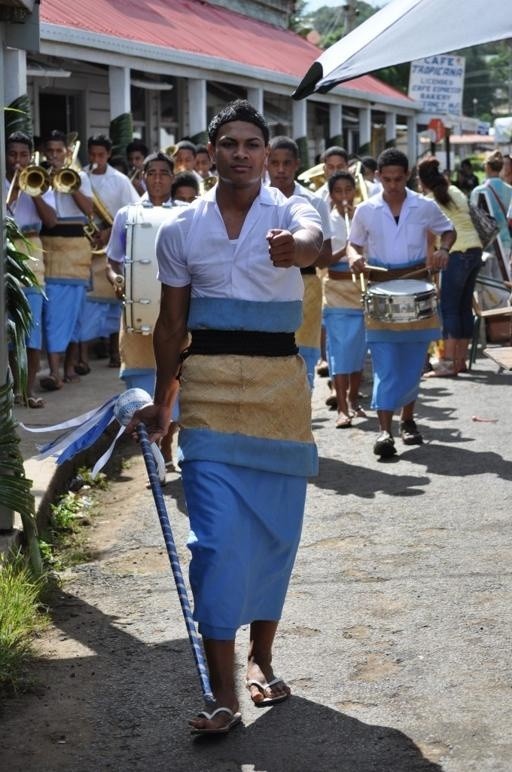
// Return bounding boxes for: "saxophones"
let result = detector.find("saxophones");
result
[85,164,115,256]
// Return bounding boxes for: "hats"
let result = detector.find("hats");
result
[480,151,504,165]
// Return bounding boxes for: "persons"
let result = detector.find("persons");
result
[130,94,326,735]
[343,149,458,459]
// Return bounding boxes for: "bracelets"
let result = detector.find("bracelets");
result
[439,246,450,252]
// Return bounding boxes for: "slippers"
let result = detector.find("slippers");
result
[188,705,241,736]
[246,674,290,705]
[27,395,44,408]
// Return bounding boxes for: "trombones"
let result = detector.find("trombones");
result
[5,130,81,218]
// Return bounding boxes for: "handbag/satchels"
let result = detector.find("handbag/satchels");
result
[468,197,501,251]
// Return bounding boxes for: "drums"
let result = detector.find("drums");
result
[362,279,439,325]
[121,205,182,337]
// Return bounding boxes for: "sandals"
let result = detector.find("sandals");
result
[349,403,366,417]
[335,407,352,427]
[455,358,466,372]
[422,359,457,378]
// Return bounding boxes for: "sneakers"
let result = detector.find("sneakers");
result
[108,353,120,367]
[373,431,397,457]
[397,419,424,446]
[40,375,63,390]
[75,359,89,375]
[63,366,79,384]
[14,393,26,407]
